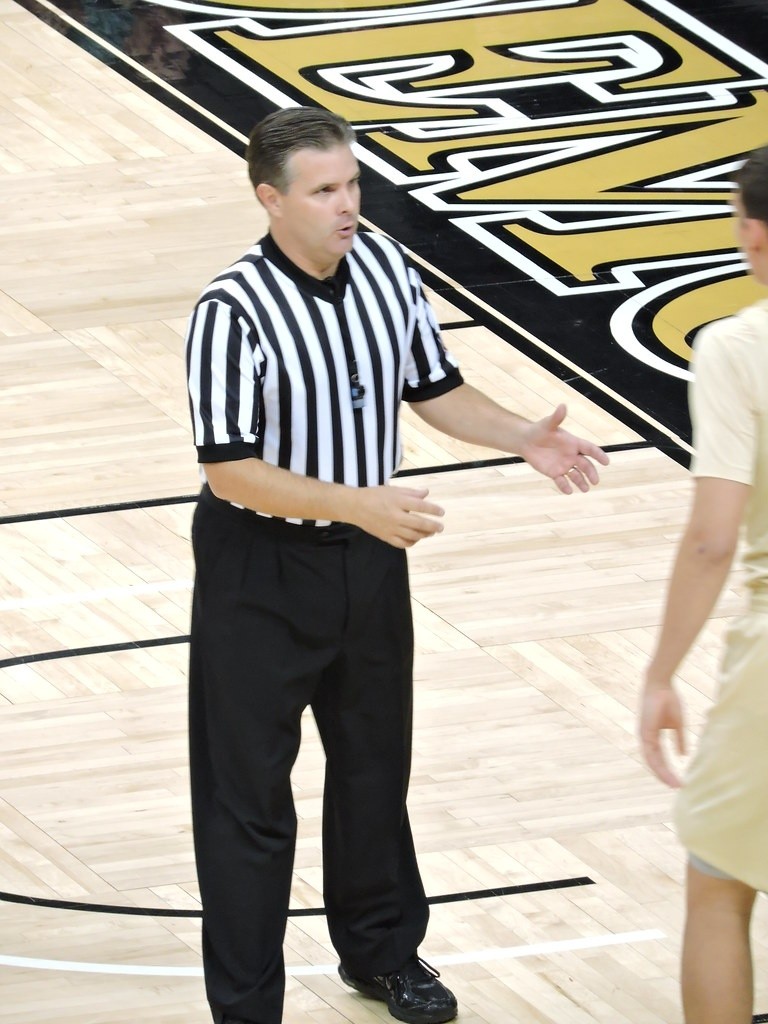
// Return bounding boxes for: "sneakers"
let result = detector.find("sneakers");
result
[337,951,461,1023]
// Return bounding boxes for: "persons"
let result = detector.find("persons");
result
[184,106,610,1024]
[639,145,768,1024]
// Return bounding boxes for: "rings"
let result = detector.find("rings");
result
[567,468,575,475]
